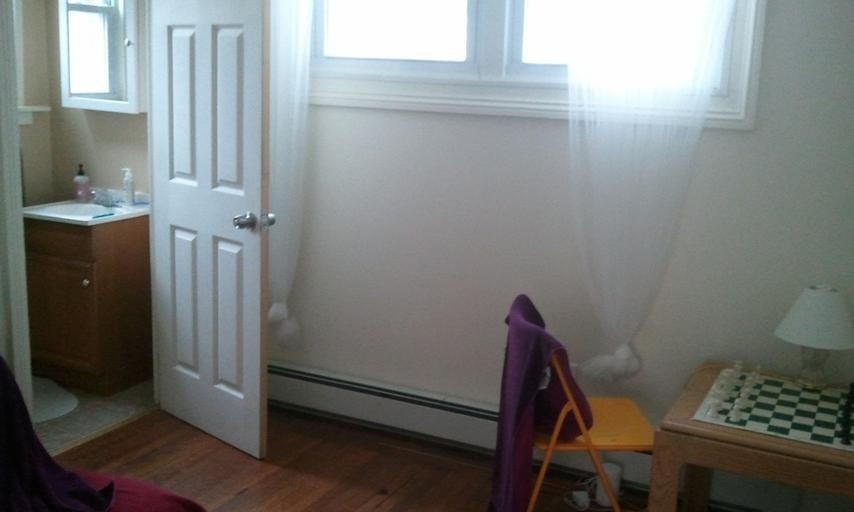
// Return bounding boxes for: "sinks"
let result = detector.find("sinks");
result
[41,201,109,218]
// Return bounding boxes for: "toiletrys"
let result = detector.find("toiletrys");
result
[73,163,90,201]
[120,167,136,207]
[121,167,134,206]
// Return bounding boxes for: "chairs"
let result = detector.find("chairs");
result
[503,294,656,511]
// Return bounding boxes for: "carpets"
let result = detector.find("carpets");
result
[29,376,81,425]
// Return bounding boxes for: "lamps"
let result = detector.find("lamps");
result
[773,284,853,392]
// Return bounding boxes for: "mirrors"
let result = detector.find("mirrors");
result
[66,0,127,101]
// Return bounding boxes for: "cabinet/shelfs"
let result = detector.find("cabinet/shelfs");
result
[57,0,147,115]
[21,217,150,398]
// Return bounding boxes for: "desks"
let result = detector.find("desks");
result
[638,361,853,510]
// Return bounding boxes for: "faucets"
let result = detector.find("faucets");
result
[89,187,114,207]
